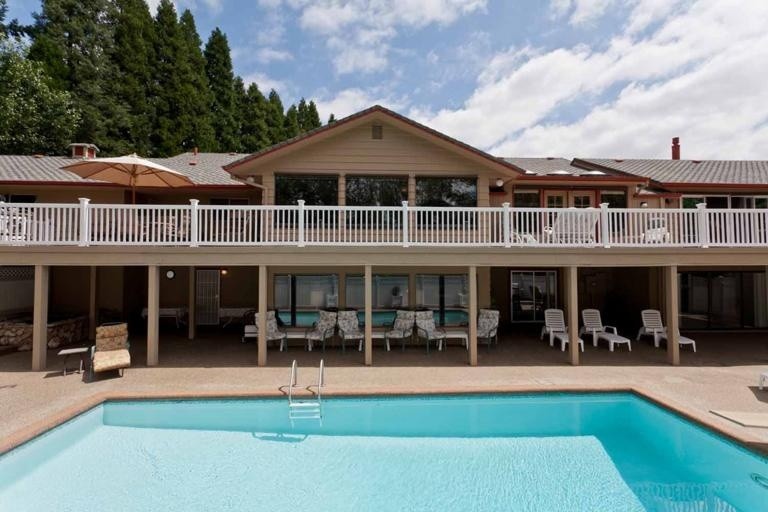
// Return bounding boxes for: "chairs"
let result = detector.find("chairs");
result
[505,207,670,244]
[100,211,194,242]
[88,323,132,383]
[540,308,697,352]
[242,308,499,354]
[758,373,768,390]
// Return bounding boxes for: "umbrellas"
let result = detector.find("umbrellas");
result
[58,152,197,204]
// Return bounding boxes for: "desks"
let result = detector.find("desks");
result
[142,307,256,329]
[58,347,88,375]
